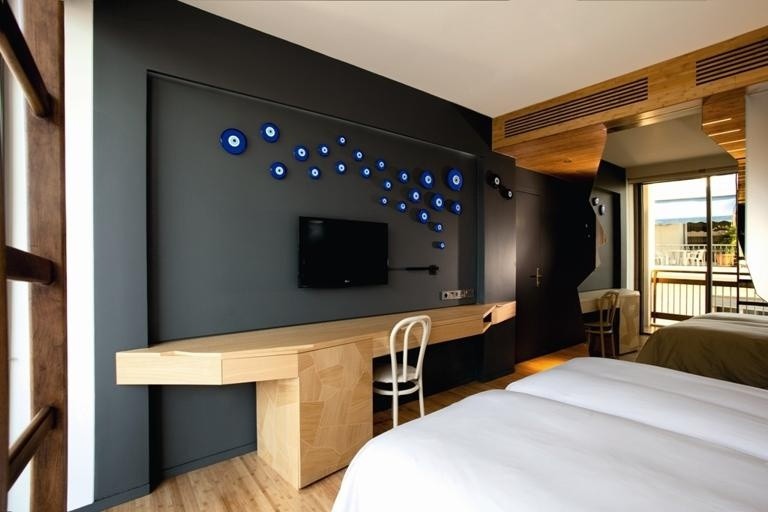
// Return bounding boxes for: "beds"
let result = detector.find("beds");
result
[334,360,768,511]
[641,314,767,388]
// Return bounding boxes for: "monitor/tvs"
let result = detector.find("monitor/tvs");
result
[299,216,389,288]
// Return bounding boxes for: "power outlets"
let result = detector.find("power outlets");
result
[441,286,476,302]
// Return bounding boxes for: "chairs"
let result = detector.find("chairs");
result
[373,315,432,428]
[583,290,620,359]
